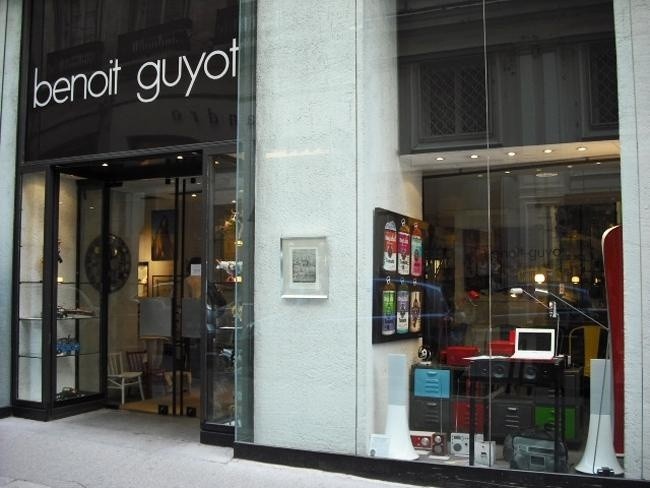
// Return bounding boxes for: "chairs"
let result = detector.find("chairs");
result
[569,325,608,377]
[126,349,151,397]
[106,350,145,405]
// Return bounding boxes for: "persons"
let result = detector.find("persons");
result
[170,255,218,380]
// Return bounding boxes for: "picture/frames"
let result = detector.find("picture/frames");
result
[279,235,330,301]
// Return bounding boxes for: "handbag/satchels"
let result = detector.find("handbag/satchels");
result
[208,281,227,318]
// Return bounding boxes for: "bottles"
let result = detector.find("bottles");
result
[382,277,423,335]
[384,216,423,278]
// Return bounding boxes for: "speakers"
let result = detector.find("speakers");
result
[410,359,584,472]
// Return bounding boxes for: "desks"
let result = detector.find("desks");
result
[469,355,565,473]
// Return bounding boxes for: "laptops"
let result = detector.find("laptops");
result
[510,327,556,360]
